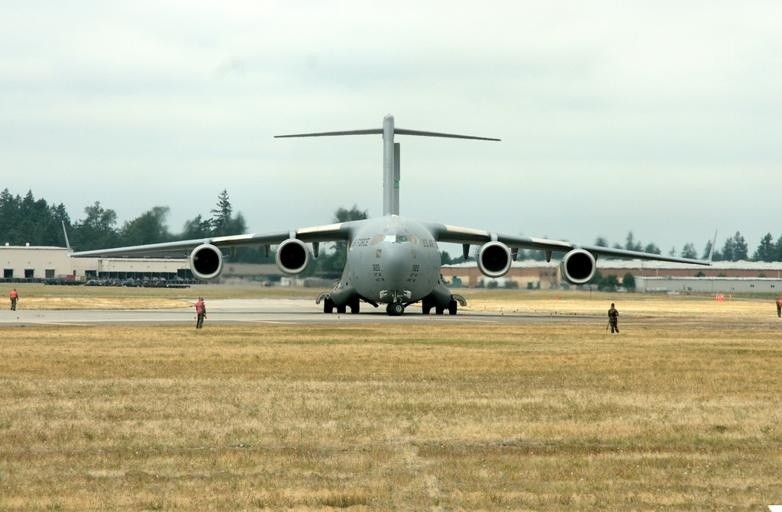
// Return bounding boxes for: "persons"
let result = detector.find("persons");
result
[10,289,19,311]
[608,304,619,333]
[196,297,206,329]
[776,297,782,317]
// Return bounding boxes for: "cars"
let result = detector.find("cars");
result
[47,275,191,290]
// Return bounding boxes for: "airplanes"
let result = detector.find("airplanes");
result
[61,115,714,318]
